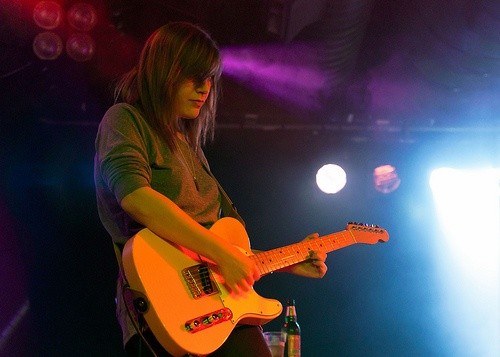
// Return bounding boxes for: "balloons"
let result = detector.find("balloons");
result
[32,0,97,61]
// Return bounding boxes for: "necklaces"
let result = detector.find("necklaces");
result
[170,128,200,192]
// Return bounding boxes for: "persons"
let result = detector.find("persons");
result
[93,21,328,357]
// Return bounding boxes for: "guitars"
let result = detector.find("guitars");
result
[120,212,390,356]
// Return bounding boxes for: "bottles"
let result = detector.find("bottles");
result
[280,298,301,357]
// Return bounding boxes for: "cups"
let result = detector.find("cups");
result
[263,332,287,357]
